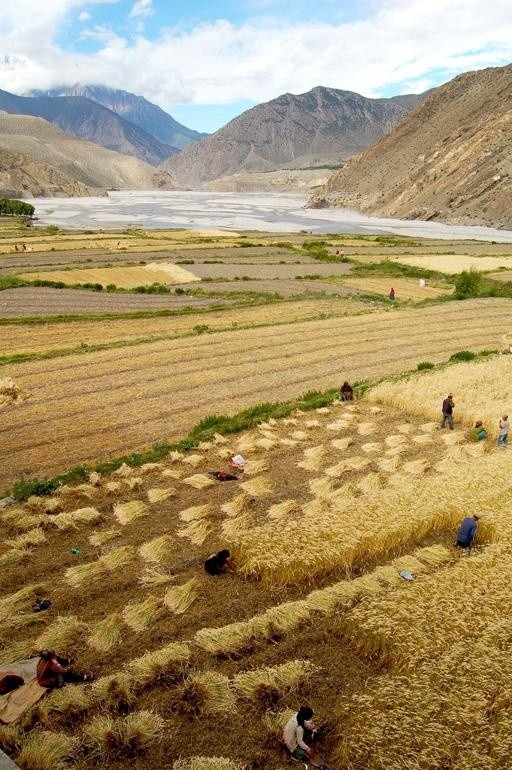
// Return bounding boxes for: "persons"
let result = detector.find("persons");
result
[390,288,394,300]
[204,549,235,576]
[441,392,455,432]
[497,414,509,448]
[473,421,487,441]
[340,381,355,401]
[453,513,480,549]
[37,647,93,688]
[282,706,319,763]
